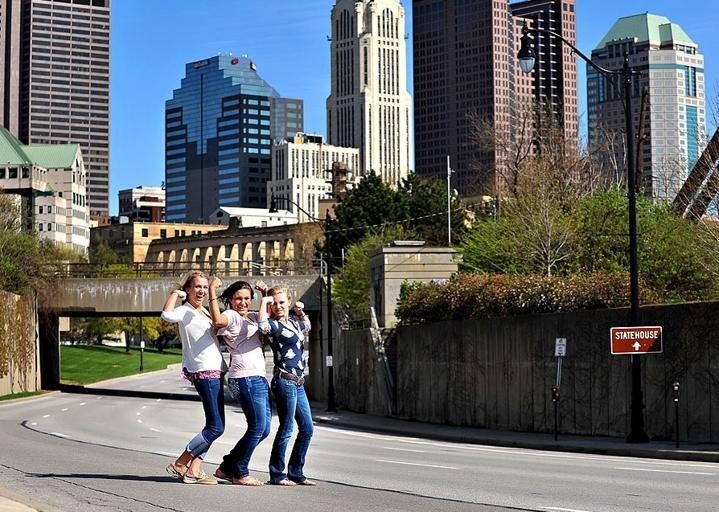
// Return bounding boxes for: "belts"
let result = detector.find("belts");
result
[275,370,305,386]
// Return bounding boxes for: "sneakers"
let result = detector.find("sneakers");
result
[166,464,218,485]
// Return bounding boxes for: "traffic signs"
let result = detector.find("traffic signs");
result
[610,325,662,355]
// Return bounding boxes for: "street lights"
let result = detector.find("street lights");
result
[268,190,341,412]
[506,16,650,444]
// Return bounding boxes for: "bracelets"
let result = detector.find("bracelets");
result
[171,291,179,296]
[207,297,216,304]
[299,312,306,319]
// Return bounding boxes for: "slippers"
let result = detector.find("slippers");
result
[213,474,264,485]
[264,479,317,486]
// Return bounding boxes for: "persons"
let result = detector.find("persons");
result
[160,269,229,486]
[257,283,319,487]
[207,271,274,487]
